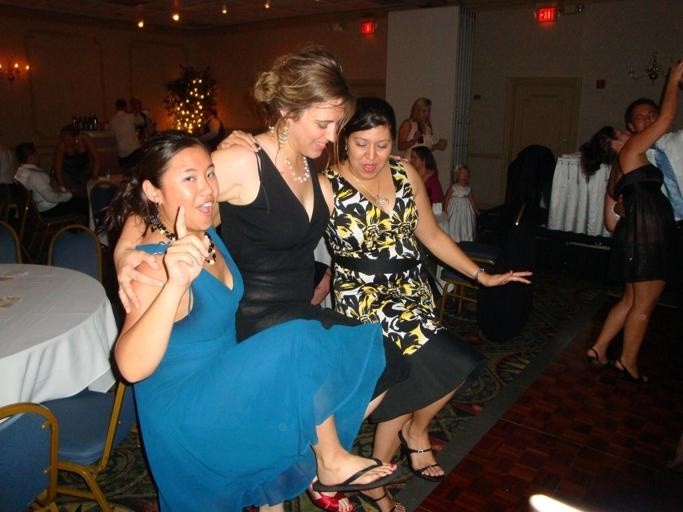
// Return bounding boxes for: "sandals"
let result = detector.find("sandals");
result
[306,429,445,511]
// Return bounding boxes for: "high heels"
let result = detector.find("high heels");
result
[612,358,652,388]
[584,346,610,377]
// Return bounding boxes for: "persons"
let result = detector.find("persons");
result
[112,42,411,511]
[108,99,155,175]
[578,60,683,386]
[444,164,481,243]
[615,97,682,289]
[112,129,405,512]
[53,124,101,193]
[325,99,534,483]
[399,98,447,210]
[200,107,225,143]
[15,144,86,221]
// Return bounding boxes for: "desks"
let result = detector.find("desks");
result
[83,130,115,153]
[540,154,613,238]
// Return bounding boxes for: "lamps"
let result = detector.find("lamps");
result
[629,54,669,85]
[0,62,29,86]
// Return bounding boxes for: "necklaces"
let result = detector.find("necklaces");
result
[352,164,388,208]
[154,218,217,269]
[269,128,311,184]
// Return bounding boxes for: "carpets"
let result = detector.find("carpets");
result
[56,261,608,509]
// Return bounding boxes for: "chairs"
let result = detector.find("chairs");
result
[438,200,528,324]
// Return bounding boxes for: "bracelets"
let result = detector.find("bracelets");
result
[474,268,485,283]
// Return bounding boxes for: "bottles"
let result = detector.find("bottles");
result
[72,112,98,131]
[106,167,111,184]
[49,165,56,177]
[416,122,424,144]
